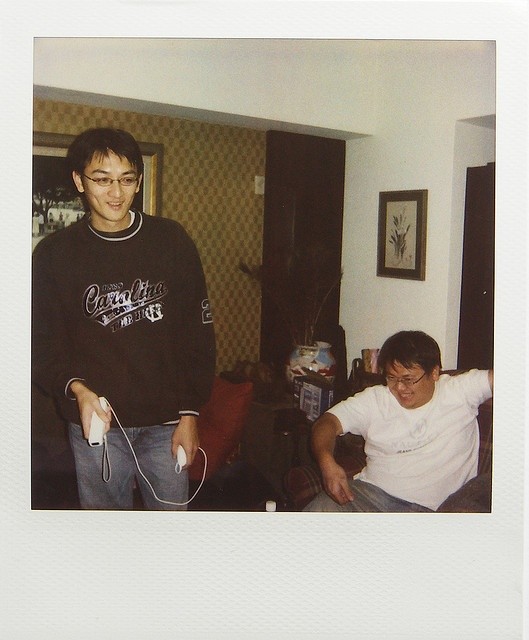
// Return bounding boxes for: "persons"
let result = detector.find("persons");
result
[299,327,494,513]
[32,123,216,510]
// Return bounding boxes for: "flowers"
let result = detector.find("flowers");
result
[389,209,411,260]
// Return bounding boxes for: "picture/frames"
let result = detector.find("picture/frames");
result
[33,131,163,252]
[377,190,427,280]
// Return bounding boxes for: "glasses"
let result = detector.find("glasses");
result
[384,369,426,386]
[75,171,139,186]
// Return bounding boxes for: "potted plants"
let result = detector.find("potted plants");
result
[239,249,349,401]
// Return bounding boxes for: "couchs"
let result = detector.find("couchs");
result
[267,369,490,512]
[32,374,268,511]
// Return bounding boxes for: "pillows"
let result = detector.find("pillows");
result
[188,376,254,480]
